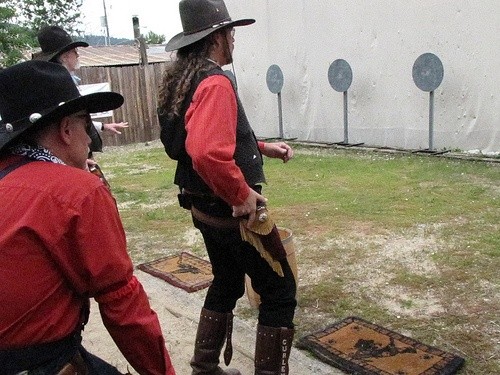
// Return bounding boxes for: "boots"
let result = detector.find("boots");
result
[189,308,240,375]
[254,324,293,375]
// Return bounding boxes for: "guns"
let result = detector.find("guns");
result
[242,185,287,262]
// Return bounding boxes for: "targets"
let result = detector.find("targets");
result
[224,70,237,92]
[326,60,353,93]
[265,65,285,94]
[411,53,444,91]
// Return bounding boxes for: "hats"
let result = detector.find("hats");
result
[31,27,90,62]
[0,60,124,153]
[164,0,254,52]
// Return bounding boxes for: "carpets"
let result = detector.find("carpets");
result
[295,316,465,375]
[136,252,214,293]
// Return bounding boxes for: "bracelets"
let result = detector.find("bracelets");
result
[101,122,104,131]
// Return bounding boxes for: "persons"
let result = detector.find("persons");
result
[0,62,175,375]
[156,0,297,375]
[31,27,129,189]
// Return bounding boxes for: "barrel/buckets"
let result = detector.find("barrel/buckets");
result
[245,226,299,310]
